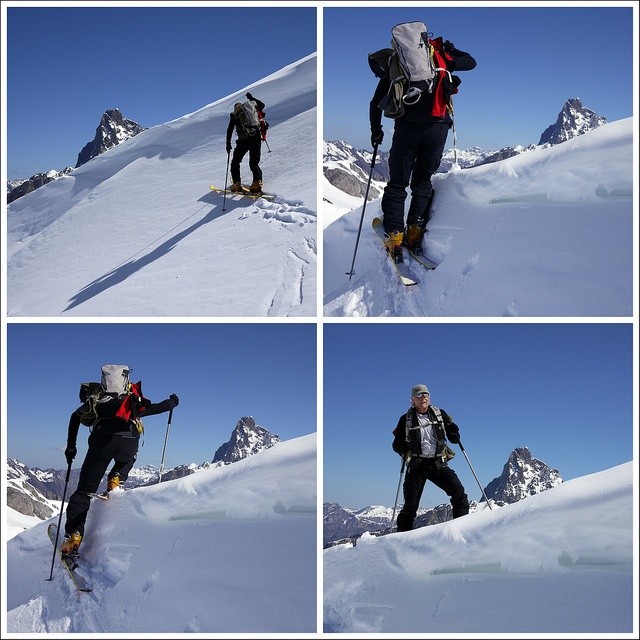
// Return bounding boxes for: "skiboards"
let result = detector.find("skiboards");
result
[48,493,109,592]
[209,178,274,200]
[372,217,438,286]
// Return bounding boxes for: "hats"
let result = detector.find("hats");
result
[412,385,430,397]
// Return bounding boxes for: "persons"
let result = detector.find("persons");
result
[390,384,469,533]
[368,19,478,256]
[224,91,269,192]
[60,364,179,555]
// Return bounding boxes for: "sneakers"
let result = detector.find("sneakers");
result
[250,180,262,192]
[230,182,242,192]
[403,223,426,255]
[60,532,83,559]
[385,231,403,254]
[103,473,125,499]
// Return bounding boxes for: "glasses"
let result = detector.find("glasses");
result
[415,394,429,397]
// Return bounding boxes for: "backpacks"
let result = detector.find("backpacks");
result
[92,365,151,433]
[391,21,437,81]
[237,100,261,137]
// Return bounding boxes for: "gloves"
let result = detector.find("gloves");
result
[401,441,410,453]
[443,40,455,55]
[169,393,179,410]
[371,129,384,147]
[448,422,459,434]
[65,446,78,465]
